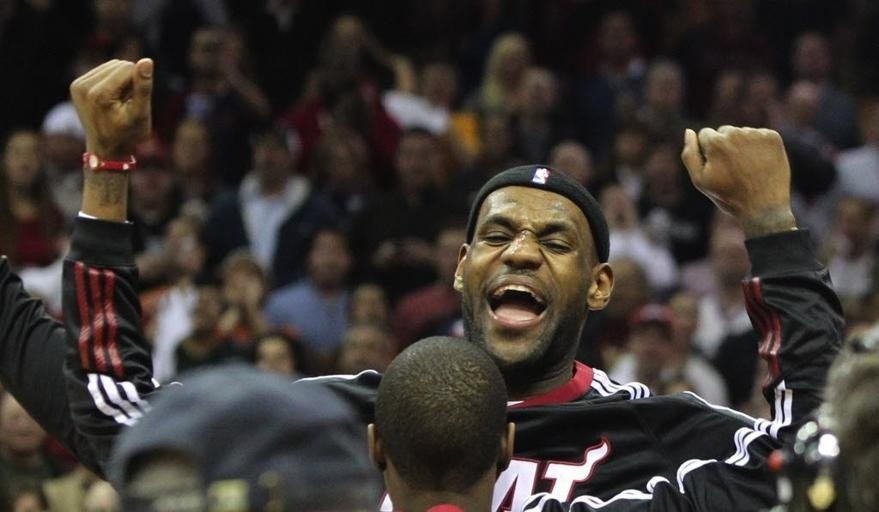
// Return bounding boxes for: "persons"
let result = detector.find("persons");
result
[3,0,879,512]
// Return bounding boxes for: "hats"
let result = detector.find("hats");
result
[466,164,609,264]
[109,367,384,512]
